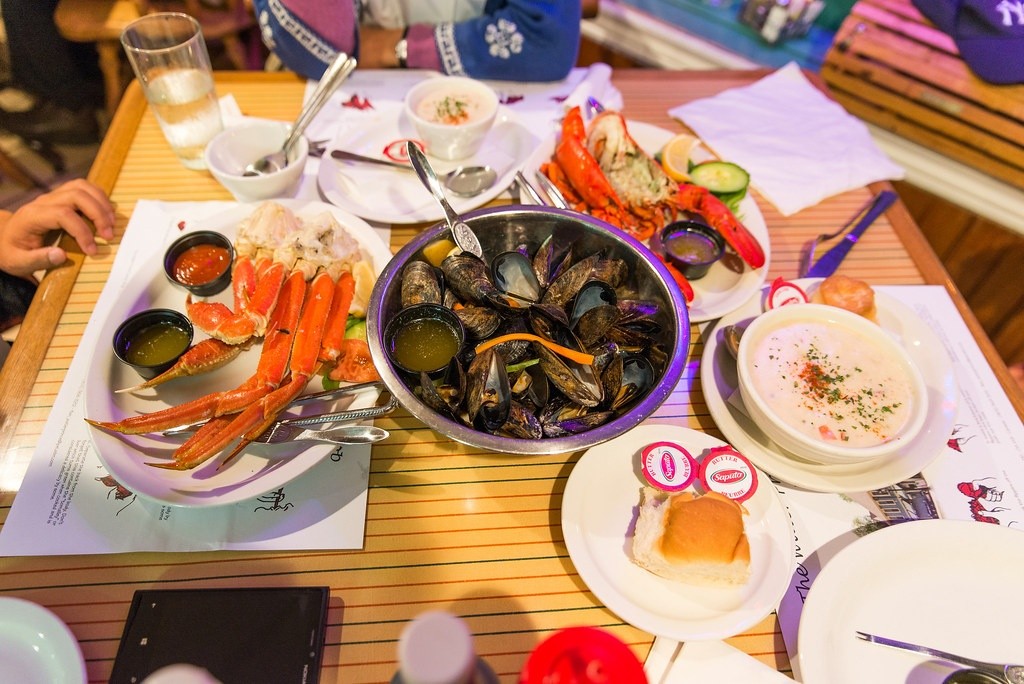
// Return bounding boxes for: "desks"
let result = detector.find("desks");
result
[0,67,1024,684]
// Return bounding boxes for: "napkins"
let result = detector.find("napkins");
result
[644,636,801,684]
[303,63,624,156]
[668,61,904,217]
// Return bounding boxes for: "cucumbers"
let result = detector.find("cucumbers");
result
[343,321,366,345]
[687,160,751,195]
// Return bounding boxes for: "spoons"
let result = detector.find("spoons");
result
[331,150,496,198]
[242,51,347,176]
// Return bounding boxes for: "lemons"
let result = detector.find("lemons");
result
[662,133,701,182]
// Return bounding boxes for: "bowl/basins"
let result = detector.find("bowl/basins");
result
[405,75,499,162]
[384,303,466,379]
[204,119,309,200]
[659,220,726,280]
[737,303,930,465]
[161,229,233,297]
[112,308,194,380]
[365,209,691,455]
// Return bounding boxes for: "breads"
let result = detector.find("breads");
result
[630,486,751,587]
[808,276,877,326]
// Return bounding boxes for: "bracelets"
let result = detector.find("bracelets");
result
[395,26,409,67]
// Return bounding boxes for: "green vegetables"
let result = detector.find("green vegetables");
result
[761,337,903,443]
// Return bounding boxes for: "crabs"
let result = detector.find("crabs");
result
[85,202,376,471]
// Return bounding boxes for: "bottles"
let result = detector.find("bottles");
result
[388,610,500,684]
[515,626,650,684]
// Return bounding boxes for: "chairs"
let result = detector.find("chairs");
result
[54,0,257,123]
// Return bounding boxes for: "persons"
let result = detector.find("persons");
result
[256,0,582,79]
[0,178,115,316]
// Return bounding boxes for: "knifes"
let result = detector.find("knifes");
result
[800,191,897,277]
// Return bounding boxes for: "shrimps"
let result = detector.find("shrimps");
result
[540,106,766,310]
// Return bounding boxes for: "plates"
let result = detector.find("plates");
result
[560,425,796,640]
[520,119,772,320]
[107,586,331,683]
[796,518,1023,683]
[700,277,959,494]
[82,217,383,509]
[316,106,544,224]
[0,597,89,684]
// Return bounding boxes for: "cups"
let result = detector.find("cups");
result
[120,12,223,170]
